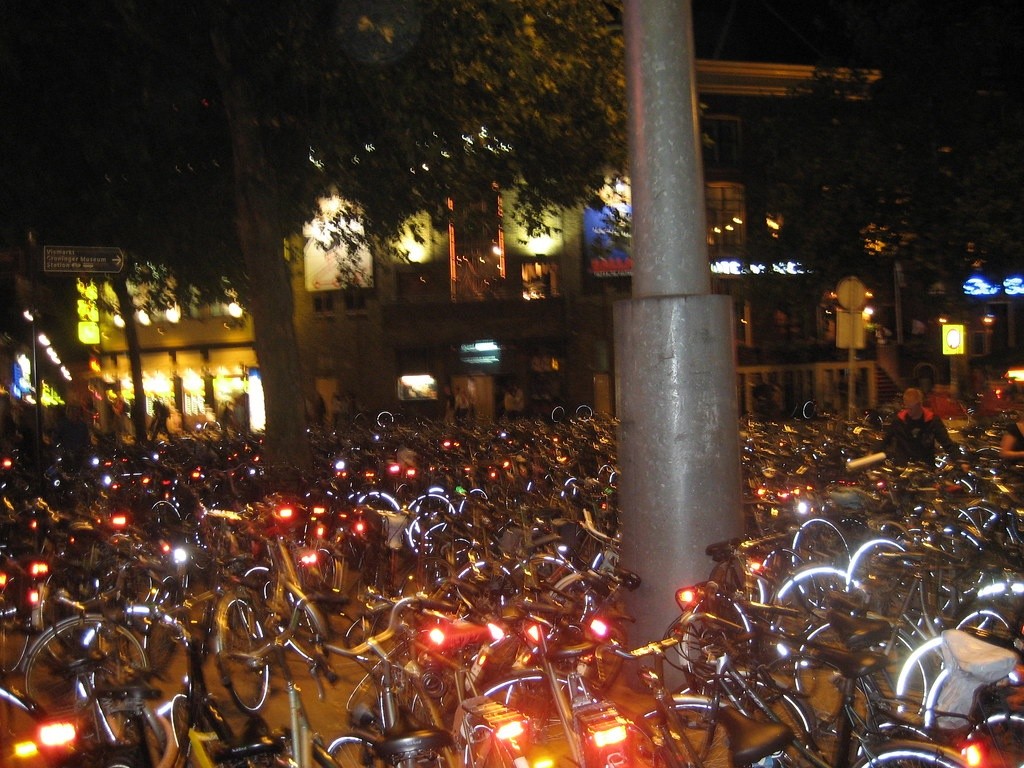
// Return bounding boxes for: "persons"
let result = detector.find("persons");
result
[998,422,1024,461]
[869,382,962,510]
[215,384,526,432]
[148,402,174,443]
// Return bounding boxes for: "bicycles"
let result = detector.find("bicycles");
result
[0,382,1024,768]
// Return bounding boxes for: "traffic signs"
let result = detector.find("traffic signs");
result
[44,243,128,272]
